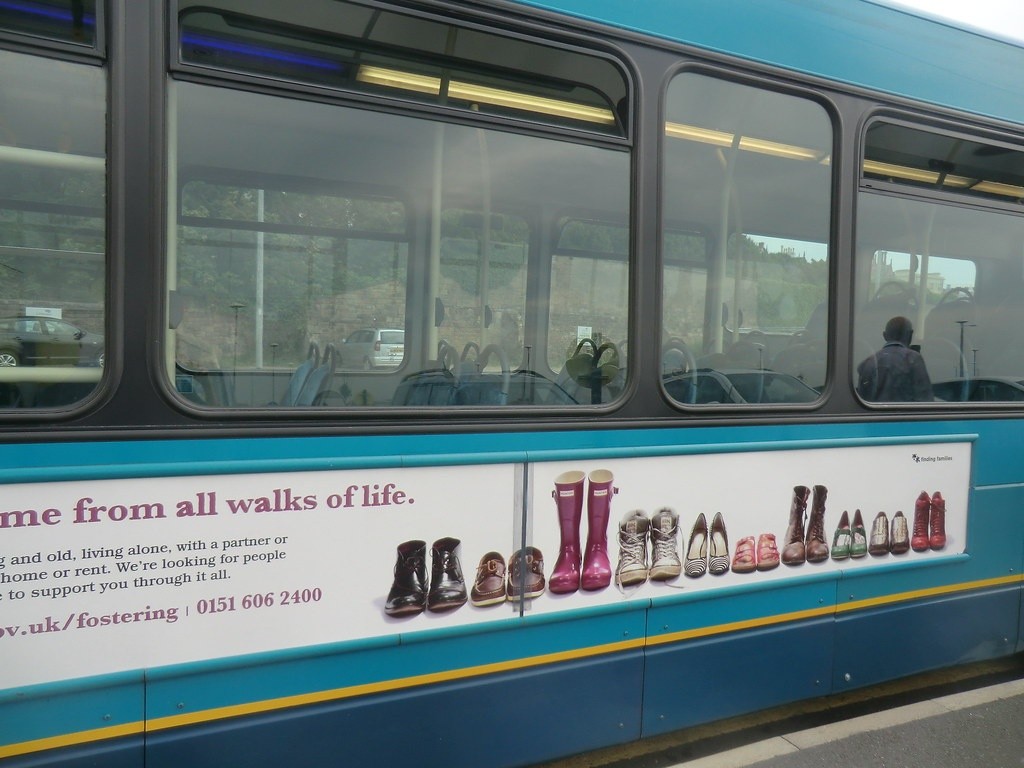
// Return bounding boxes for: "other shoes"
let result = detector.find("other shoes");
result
[706,511,730,573]
[684,512,708,577]
[888,510,910,553]
[831,511,851,558]
[850,509,869,557]
[868,511,889,554]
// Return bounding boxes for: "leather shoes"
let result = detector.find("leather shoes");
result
[385,538,428,614]
[427,537,467,609]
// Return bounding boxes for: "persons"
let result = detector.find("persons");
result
[857,317,934,402]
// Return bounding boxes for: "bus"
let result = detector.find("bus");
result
[0,0,1023,768]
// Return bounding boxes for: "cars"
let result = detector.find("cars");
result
[0,315,105,369]
[329,329,404,371]
[932,375,1024,404]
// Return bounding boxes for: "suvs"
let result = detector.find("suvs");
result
[662,367,820,407]
[386,368,582,408]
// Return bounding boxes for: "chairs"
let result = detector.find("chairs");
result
[394,341,515,406]
[707,281,987,403]
[541,335,700,405]
[279,341,338,408]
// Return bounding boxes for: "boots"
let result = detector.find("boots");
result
[804,485,829,561]
[781,485,811,564]
[550,470,585,592]
[930,491,947,550]
[581,469,619,589]
[911,491,931,552]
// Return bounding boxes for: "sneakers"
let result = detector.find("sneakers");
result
[649,506,685,578]
[505,546,544,601]
[614,508,652,593]
[470,551,508,606]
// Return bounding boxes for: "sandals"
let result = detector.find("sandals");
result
[756,533,780,570]
[732,535,755,572]
